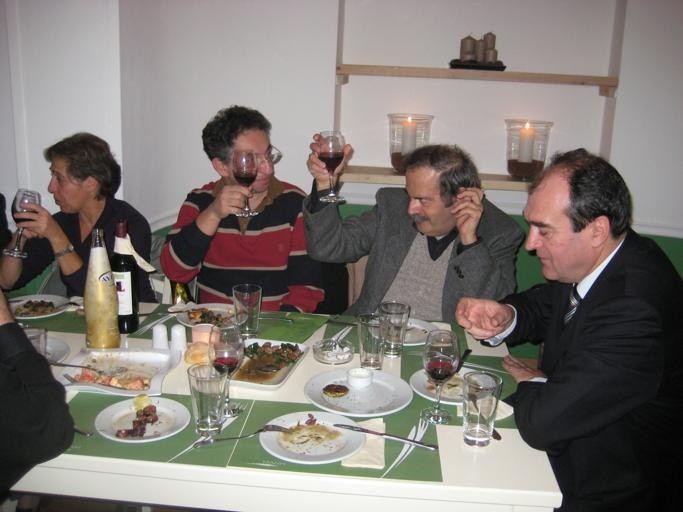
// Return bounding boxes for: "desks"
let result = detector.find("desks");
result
[5,294,567,511]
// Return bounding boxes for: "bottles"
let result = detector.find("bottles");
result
[460,31,498,67]
[84,228,120,349]
[110,223,141,334]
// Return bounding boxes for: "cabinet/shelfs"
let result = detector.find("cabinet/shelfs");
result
[334,0,630,194]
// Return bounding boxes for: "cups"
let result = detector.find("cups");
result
[379,299,410,356]
[503,116,553,182]
[187,361,228,436]
[232,284,262,337]
[353,312,387,371]
[459,369,502,448]
[386,111,433,174]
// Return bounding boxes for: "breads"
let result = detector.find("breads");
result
[185,343,215,364]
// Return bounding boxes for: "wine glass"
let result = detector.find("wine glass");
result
[1,187,40,260]
[231,149,259,217]
[419,326,460,427]
[210,327,247,417]
[317,128,344,204]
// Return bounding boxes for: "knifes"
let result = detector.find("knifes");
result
[332,422,438,450]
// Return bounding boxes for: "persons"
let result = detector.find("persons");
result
[160,105,326,314]
[0,132,159,302]
[302,134,524,322]
[0,289,74,506]
[455,149,683,512]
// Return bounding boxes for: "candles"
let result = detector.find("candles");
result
[518,121,535,163]
[400,116,417,155]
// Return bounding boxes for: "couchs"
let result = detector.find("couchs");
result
[145,199,681,358]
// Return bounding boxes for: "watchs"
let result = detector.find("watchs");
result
[55,243,74,257]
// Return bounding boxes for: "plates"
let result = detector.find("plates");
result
[94,397,191,444]
[27,330,73,366]
[4,294,72,319]
[257,409,366,464]
[54,348,183,397]
[303,367,413,419]
[367,317,439,346]
[177,301,248,328]
[409,365,474,406]
[228,337,308,393]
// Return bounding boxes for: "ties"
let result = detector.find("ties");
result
[563,286,583,325]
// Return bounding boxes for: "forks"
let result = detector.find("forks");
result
[193,423,290,447]
